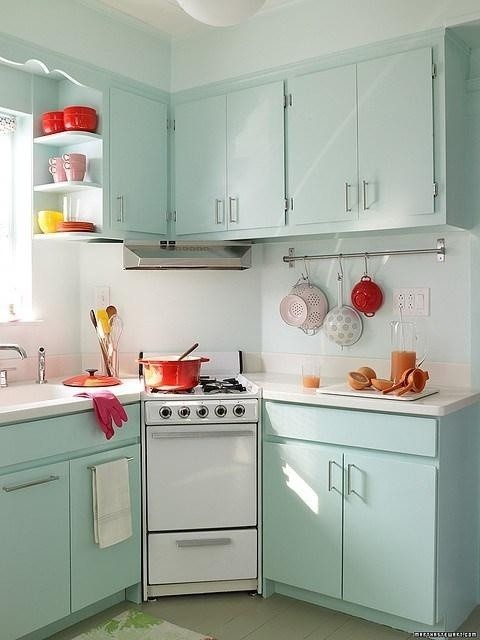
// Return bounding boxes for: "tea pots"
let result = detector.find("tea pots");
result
[389,320,430,387]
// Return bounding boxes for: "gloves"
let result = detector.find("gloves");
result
[73,390,127,439]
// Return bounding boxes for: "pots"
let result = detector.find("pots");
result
[135,353,210,393]
[323,272,364,349]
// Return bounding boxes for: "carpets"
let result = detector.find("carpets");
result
[68,607,216,640]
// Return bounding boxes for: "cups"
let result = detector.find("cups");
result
[47,153,87,183]
[101,350,119,379]
[300,362,321,389]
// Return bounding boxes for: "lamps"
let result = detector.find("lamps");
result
[177,0,268,28]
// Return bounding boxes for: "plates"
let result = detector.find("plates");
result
[56,221,95,233]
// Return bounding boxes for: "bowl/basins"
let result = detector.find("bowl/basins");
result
[37,211,65,234]
[350,274,382,317]
[348,367,393,392]
[41,106,97,135]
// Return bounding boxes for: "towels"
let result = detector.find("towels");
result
[92,457,133,549]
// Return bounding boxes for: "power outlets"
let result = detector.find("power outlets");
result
[405,287,416,315]
[96,287,110,307]
[393,288,405,315]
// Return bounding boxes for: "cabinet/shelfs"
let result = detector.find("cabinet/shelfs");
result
[107,68,171,241]
[261,398,480,640]
[33,130,103,240]
[171,65,288,243]
[288,26,470,241]
[0,403,142,640]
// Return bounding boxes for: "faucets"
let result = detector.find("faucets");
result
[37,346,46,383]
[0,343,27,359]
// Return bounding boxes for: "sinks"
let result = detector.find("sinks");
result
[0,385,91,412]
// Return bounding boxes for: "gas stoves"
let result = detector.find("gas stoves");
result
[137,372,261,425]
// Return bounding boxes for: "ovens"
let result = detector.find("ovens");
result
[138,425,261,601]
